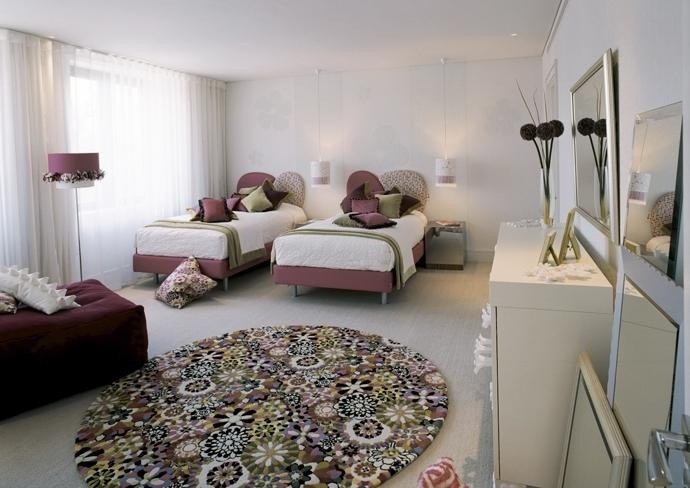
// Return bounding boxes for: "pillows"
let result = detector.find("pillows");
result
[332,183,421,230]
[0,263,82,314]
[660,223,672,237]
[0,290,27,314]
[155,255,217,309]
[186,178,289,223]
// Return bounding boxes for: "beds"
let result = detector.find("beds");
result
[645,192,675,263]
[133,170,308,292]
[273,170,430,305]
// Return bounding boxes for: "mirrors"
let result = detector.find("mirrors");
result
[568,48,620,246]
[607,244,684,488]
[623,101,683,274]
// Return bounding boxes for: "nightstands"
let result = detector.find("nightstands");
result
[424,220,468,270]
[294,219,324,229]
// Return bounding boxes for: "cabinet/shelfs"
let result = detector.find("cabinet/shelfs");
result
[473,220,615,488]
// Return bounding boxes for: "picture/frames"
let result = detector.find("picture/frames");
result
[559,208,581,263]
[556,351,632,488]
[538,230,560,266]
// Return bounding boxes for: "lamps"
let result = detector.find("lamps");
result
[628,118,651,206]
[311,68,331,186]
[42,153,106,282]
[435,57,456,188]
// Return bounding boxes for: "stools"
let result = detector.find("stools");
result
[0,279,149,423]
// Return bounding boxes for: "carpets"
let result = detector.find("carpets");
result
[74,325,448,488]
